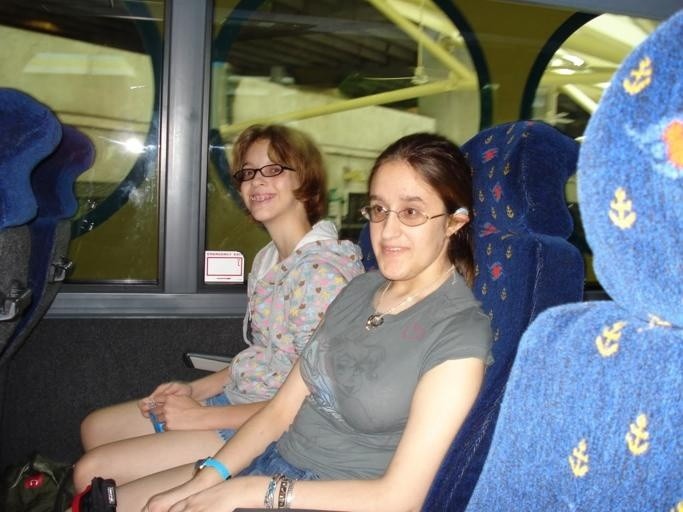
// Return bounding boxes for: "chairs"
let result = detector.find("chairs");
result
[0,87,98,367]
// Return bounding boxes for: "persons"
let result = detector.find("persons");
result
[71,121,369,502]
[110,132,496,512]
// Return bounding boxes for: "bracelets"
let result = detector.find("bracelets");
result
[263,472,284,510]
[277,477,292,510]
[196,457,231,482]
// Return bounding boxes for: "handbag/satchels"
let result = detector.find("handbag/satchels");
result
[0,450,73,512]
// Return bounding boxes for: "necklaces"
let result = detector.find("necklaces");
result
[365,267,454,332]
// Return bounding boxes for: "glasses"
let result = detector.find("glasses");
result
[359,200,455,229]
[232,163,297,183]
